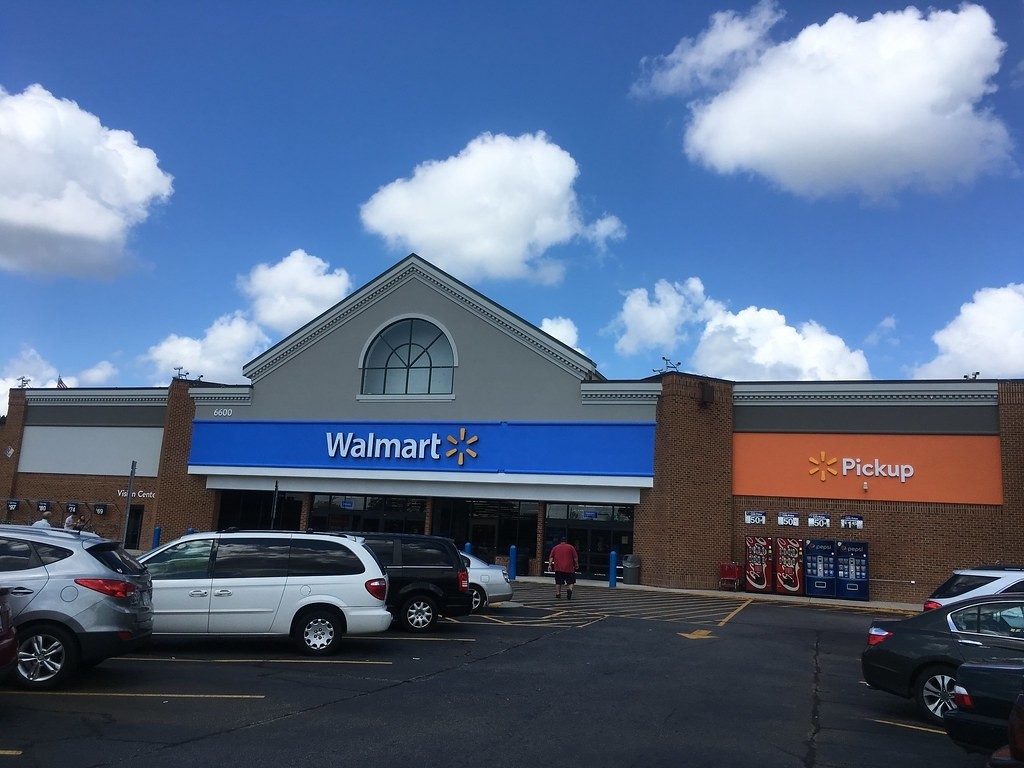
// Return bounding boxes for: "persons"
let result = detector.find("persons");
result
[64,512,85,530]
[548,536,578,600]
[32,511,52,527]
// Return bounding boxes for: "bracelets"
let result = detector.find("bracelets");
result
[549,565,552,566]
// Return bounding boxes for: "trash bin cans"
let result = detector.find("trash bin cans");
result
[622,554,641,585]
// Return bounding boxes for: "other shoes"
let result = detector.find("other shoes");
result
[555,594,561,598]
[567,589,572,599]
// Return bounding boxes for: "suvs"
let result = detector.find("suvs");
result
[0,521,155,691]
[134,528,393,655]
[922,566,1024,635]
[342,533,474,633]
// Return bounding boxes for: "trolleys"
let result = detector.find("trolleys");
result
[718,561,745,592]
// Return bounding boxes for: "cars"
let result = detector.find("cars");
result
[404,547,513,614]
[941,655,1024,768]
[860,593,1024,725]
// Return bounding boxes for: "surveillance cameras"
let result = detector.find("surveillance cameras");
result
[653,368,663,372]
[175,366,203,378]
[972,372,980,375]
[675,362,681,366]
[662,357,670,362]
[17,376,31,387]
[963,374,969,378]
